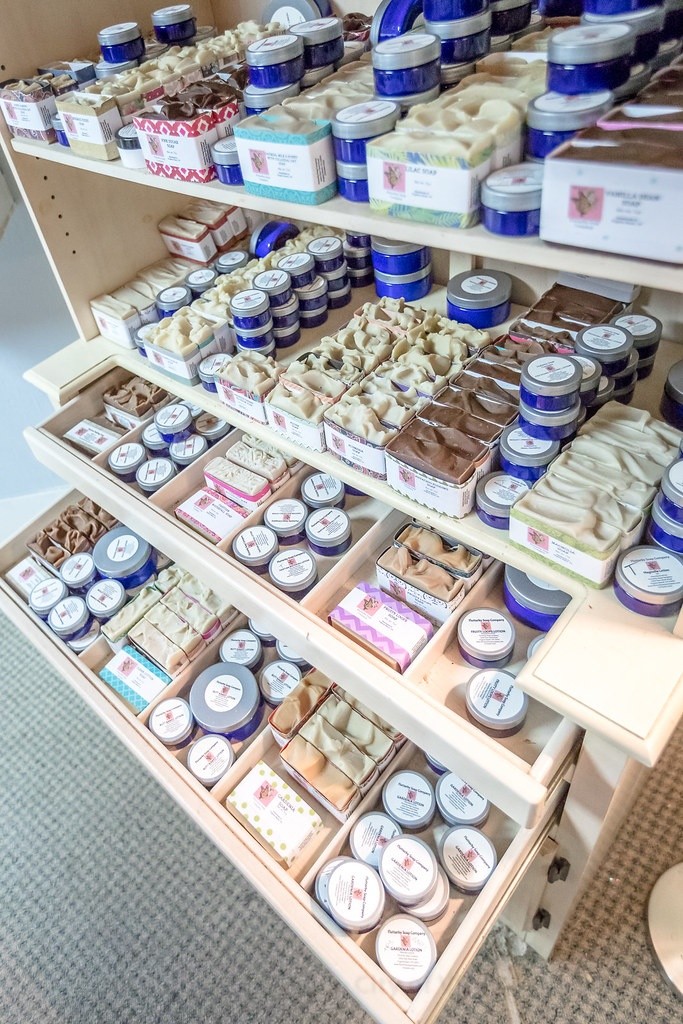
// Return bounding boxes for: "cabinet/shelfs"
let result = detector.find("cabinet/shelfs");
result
[0,0,682,1024]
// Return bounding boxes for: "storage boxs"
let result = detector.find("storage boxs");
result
[89,197,681,592]
[233,53,382,207]
[541,56,682,266]
[1,19,242,181]
[364,16,579,228]
[4,498,418,895]
